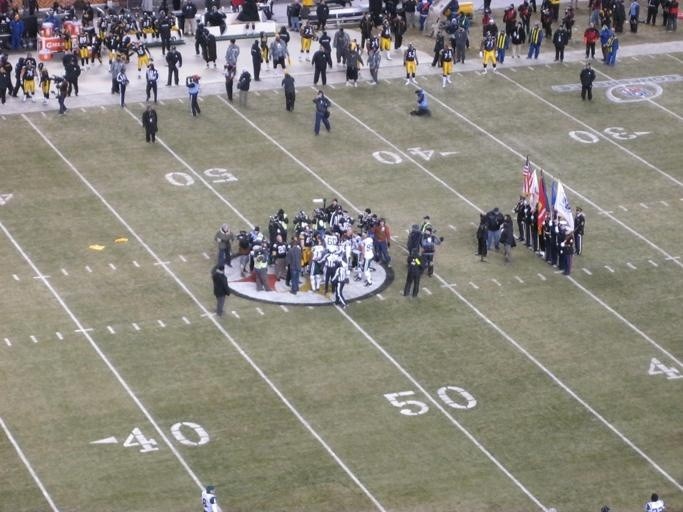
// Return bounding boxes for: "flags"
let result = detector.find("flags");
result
[523,162,574,233]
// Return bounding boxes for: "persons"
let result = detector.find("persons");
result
[202,485,217,512]
[475,196,584,277]
[358,0,472,118]
[644,493,664,512]
[478,1,679,101]
[211,196,444,315]
[192,1,365,135]
[602,506,610,512]
[0,1,203,142]
